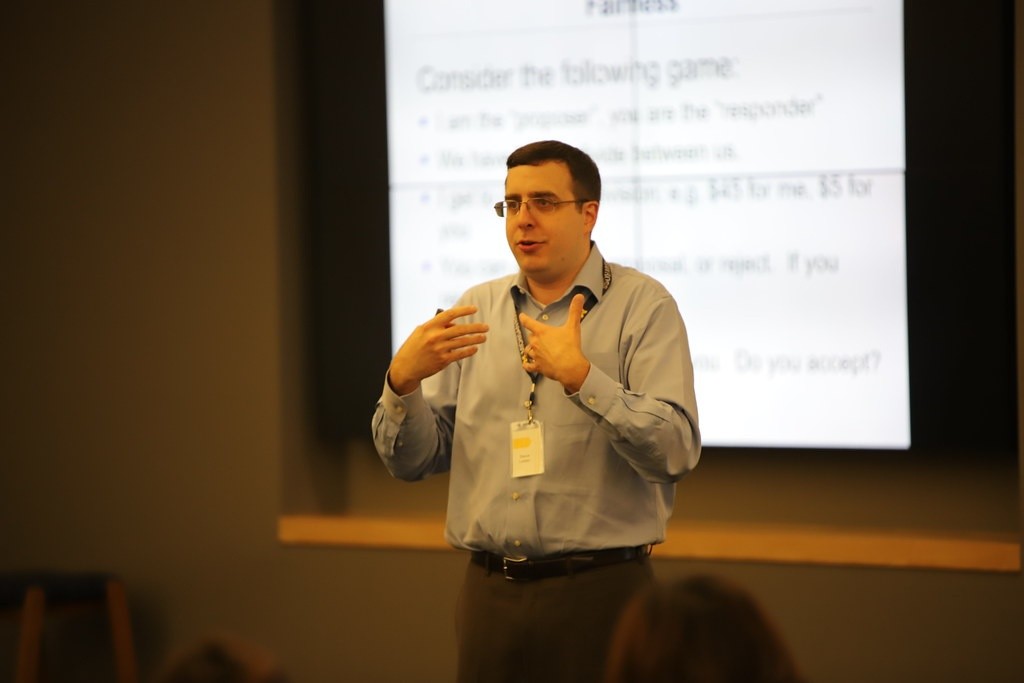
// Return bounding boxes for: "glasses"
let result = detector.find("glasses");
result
[494,198,587,217]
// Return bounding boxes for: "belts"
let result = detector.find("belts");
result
[470,542,651,582]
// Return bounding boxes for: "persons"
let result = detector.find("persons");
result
[373,141,701,683]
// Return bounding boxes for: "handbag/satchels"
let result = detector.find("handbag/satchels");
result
[0,569,170,683]
